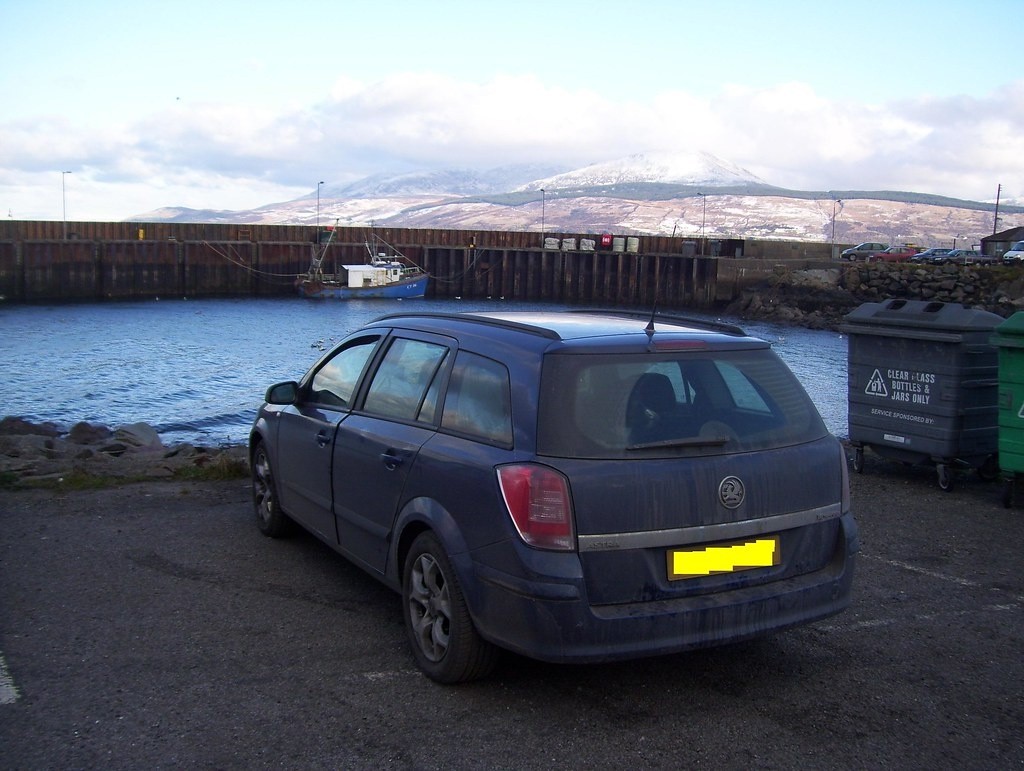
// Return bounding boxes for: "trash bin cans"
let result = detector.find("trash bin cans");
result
[990,312,1024,509]
[838,298,1006,491]
[681,241,698,255]
[708,239,723,257]
[832,247,840,259]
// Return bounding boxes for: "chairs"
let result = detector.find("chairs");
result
[618,373,687,447]
[400,357,468,421]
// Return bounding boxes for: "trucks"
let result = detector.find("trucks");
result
[944,249,1008,268]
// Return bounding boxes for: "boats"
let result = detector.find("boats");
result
[294,220,429,300]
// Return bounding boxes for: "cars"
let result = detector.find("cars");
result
[247,307,852,686]
[870,246,917,262]
[841,242,888,261]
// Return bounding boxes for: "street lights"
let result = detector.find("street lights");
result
[317,181,324,244]
[541,188,546,247]
[832,198,841,260]
[62,171,72,239]
[697,192,705,254]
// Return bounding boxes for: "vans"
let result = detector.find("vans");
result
[1003,241,1024,265]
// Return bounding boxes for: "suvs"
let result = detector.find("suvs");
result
[930,249,978,266]
[910,248,952,263]
